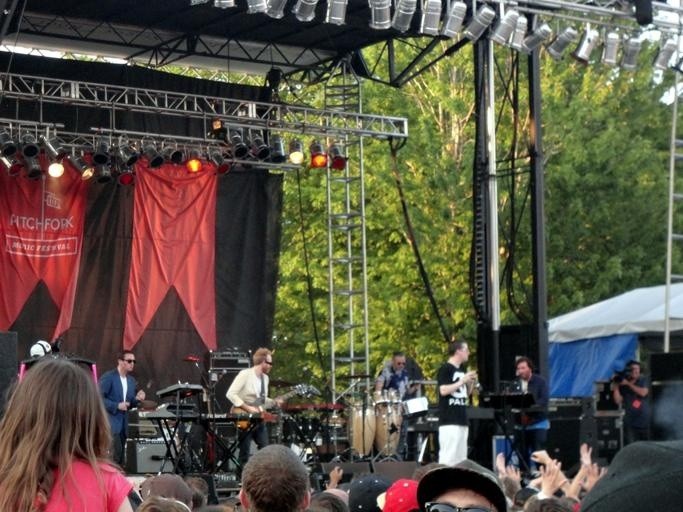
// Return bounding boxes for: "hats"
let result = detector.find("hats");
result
[416,457,508,512]
[346,472,396,512]
[513,487,540,506]
[375,478,420,512]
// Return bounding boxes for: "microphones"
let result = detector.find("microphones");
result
[211,369,227,389]
[151,454,171,461]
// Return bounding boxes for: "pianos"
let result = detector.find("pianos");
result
[138,409,229,423]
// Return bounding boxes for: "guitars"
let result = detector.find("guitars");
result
[231,383,322,431]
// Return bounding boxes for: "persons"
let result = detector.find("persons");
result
[437,339,478,467]
[0,357,135,512]
[226,346,284,484]
[508,356,550,478]
[612,359,650,445]
[374,351,424,462]
[139,441,612,512]
[98,349,146,476]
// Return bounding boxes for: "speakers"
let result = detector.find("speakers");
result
[549,416,597,459]
[370,461,418,478]
[0,331,17,417]
[319,460,371,482]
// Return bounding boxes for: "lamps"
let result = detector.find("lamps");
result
[246,132,271,162]
[0,119,203,189]
[185,0,678,74]
[288,140,305,166]
[205,141,229,175]
[327,142,349,172]
[228,130,249,160]
[309,139,328,169]
[269,132,287,165]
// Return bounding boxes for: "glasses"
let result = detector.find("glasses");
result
[423,500,494,512]
[123,359,136,364]
[397,362,406,366]
[265,360,274,367]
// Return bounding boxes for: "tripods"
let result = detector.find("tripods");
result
[333,404,403,463]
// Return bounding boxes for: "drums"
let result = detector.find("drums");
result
[372,389,400,407]
[401,398,429,417]
[350,402,403,458]
